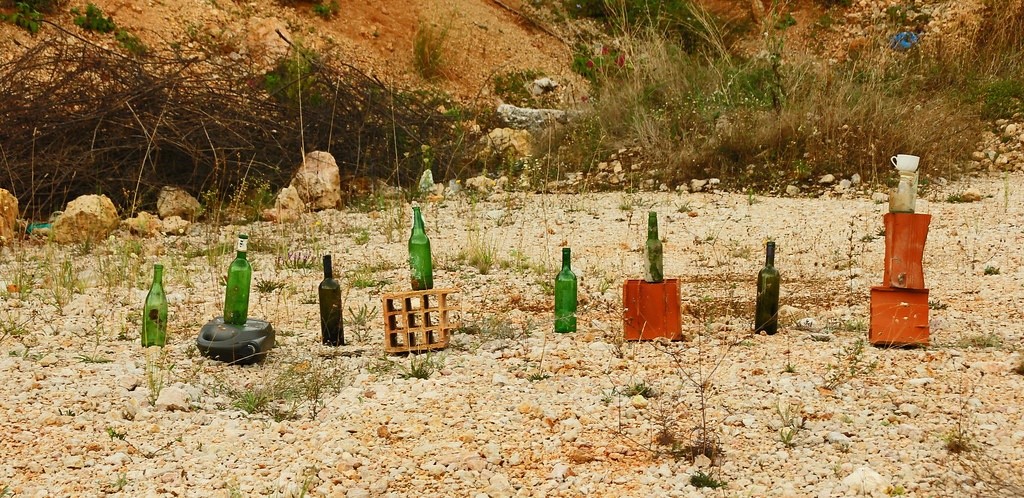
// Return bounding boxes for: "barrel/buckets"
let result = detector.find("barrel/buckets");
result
[196,316,276,366]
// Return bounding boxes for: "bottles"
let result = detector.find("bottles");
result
[140,264,168,348]
[407,206,434,291]
[554,247,578,334]
[643,211,663,284]
[317,254,345,347]
[223,234,252,325]
[754,241,781,336]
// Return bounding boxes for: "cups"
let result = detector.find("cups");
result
[889,172,918,213]
[890,153,920,173]
